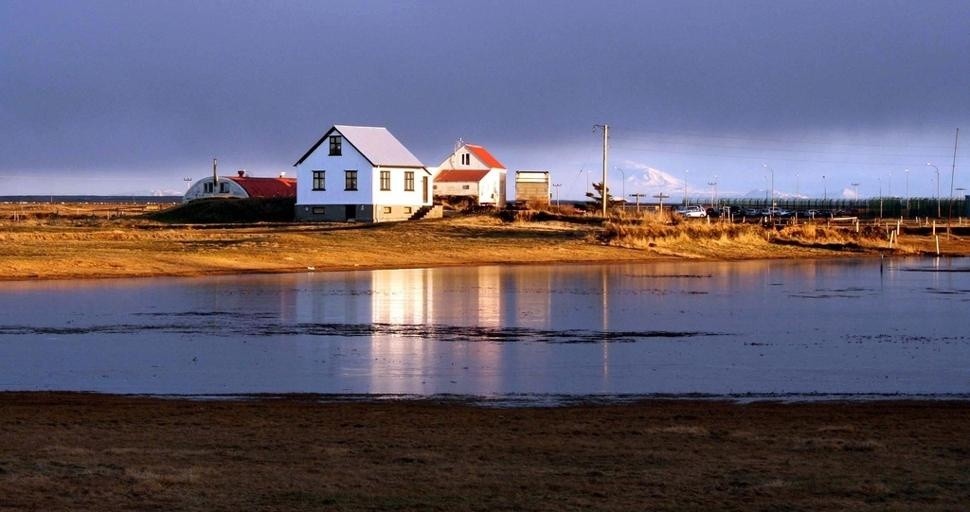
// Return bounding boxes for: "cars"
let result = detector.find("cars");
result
[678,203,858,218]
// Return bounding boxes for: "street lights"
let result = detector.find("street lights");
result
[684,162,942,220]
[616,166,626,210]
